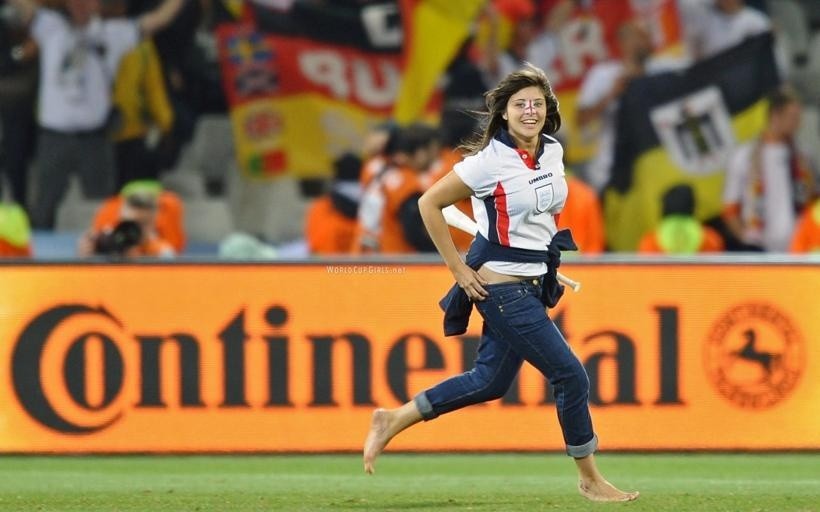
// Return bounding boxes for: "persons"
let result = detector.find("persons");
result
[2,0,820,259]
[363,62,641,502]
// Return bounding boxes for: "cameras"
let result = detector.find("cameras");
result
[94,219,139,254]
